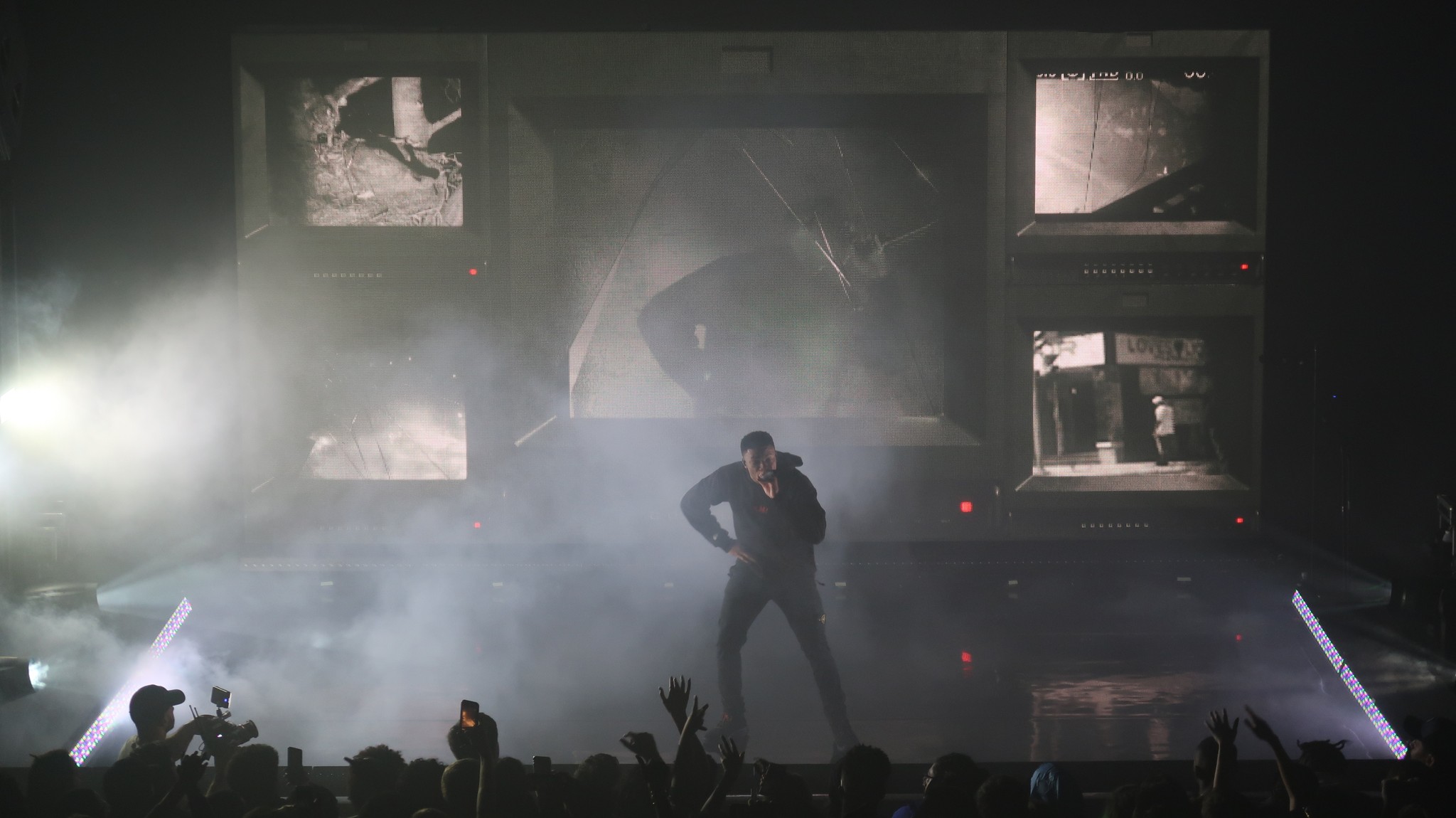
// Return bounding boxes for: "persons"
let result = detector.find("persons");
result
[680,431,860,761]
[1151,395,1175,465]
[0,686,1456,817]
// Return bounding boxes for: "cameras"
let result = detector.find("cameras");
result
[193,686,259,754]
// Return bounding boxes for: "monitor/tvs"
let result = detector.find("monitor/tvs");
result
[290,61,478,486]
[1024,57,1254,494]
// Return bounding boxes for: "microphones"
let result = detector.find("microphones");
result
[759,470,777,482]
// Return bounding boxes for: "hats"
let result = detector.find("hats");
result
[129,685,186,724]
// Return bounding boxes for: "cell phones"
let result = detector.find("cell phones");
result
[287,747,303,776]
[532,756,551,773]
[460,700,479,732]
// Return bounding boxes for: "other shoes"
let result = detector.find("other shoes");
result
[830,732,861,765]
[701,713,748,752]
